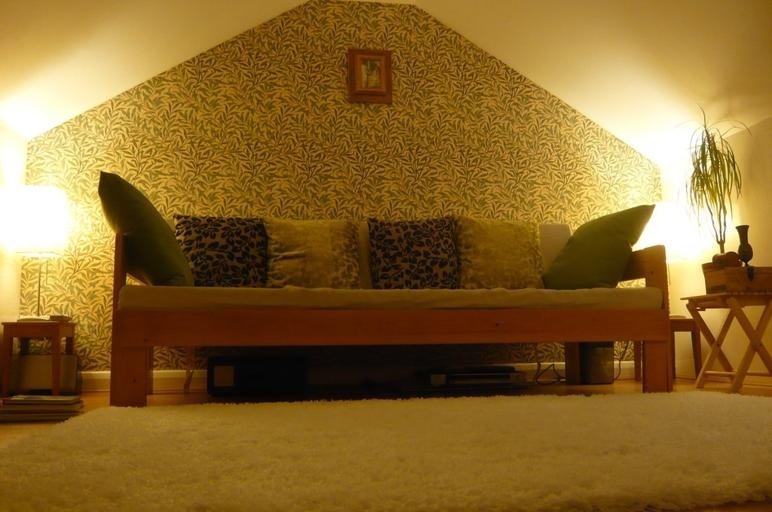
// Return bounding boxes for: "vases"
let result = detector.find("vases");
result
[735,225,752,267]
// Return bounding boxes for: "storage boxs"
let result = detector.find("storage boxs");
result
[706,266,772,295]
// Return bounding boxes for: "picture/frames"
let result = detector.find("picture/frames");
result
[347,48,392,104]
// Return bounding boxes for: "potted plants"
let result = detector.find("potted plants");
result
[686,103,752,294]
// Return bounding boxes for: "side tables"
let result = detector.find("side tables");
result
[0,321,77,396]
[679,292,772,393]
[635,316,702,382]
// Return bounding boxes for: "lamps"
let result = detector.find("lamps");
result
[2,185,69,322]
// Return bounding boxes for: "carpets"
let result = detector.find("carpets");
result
[1,385,770,511]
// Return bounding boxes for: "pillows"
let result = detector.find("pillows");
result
[366,215,459,287]
[263,216,359,288]
[456,213,546,288]
[170,212,268,288]
[97,170,195,288]
[539,204,657,288]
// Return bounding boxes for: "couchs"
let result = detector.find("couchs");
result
[109,233,675,407]
